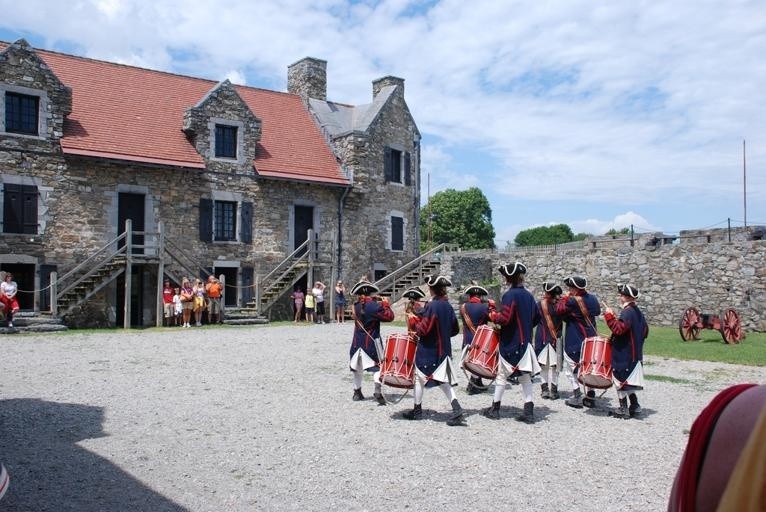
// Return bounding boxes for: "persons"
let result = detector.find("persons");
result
[547,270,602,411]
[303,289,317,323]
[0,272,19,329]
[601,281,649,418]
[334,280,347,324]
[291,285,304,324]
[532,280,565,401]
[158,269,225,328]
[483,261,537,425]
[401,271,470,427]
[458,281,493,396]
[400,286,425,339]
[311,280,328,325]
[348,276,395,406]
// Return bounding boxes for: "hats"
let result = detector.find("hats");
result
[350,262,639,299]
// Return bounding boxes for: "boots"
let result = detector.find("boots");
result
[352,375,642,427]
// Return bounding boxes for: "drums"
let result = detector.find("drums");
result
[578,336,613,388]
[467,325,500,380]
[379,333,417,390]
